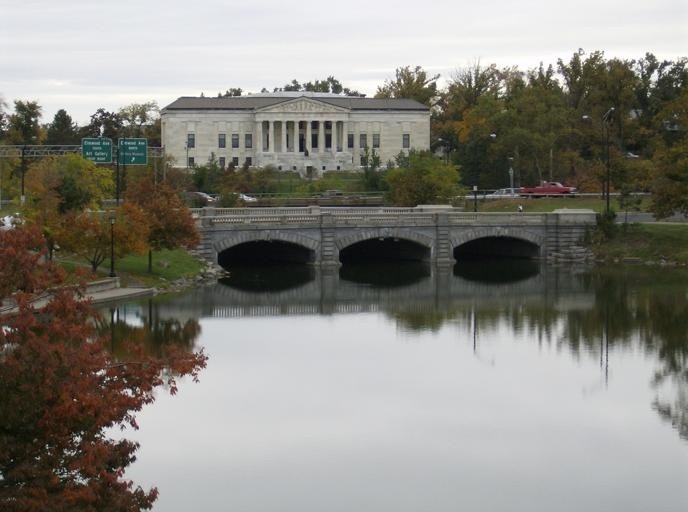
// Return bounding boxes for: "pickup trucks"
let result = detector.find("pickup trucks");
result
[519,181,577,198]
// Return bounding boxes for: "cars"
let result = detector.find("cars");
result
[215,192,257,204]
[486,187,519,197]
[187,191,217,206]
[626,152,639,159]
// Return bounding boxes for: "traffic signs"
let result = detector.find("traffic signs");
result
[81,138,112,164]
[118,137,148,166]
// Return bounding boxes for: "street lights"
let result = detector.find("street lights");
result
[20,136,37,205]
[438,138,449,161]
[581,107,616,199]
